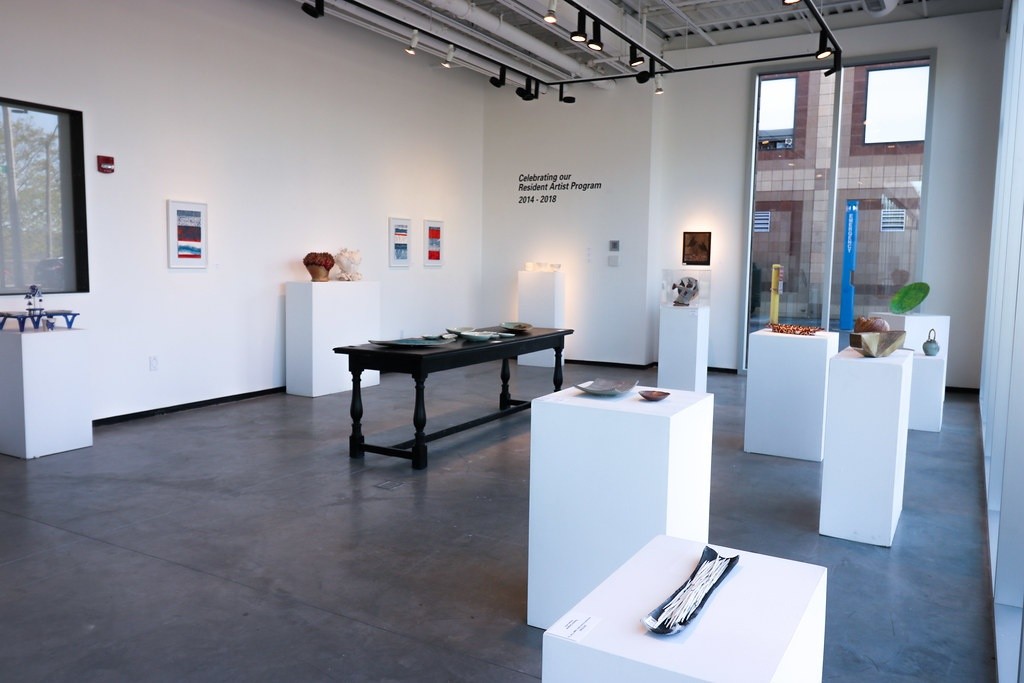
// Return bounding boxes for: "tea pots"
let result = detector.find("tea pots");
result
[923,328,940,356]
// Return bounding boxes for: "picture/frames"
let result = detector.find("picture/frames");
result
[683,232,711,265]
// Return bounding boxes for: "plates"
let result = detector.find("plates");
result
[368,320,533,348]
[889,282,929,314]
[573,376,669,401]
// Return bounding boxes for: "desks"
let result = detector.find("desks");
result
[334,324,573,469]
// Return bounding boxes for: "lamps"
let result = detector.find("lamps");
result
[490,68,506,87]
[629,46,664,94]
[782,0,832,59]
[515,79,542,101]
[559,84,575,103]
[441,44,455,68]
[300,0,325,18]
[404,30,421,55]
[543,0,604,51]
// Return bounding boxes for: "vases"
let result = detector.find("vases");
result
[306,264,328,282]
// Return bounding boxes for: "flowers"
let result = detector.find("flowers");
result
[302,252,335,270]
